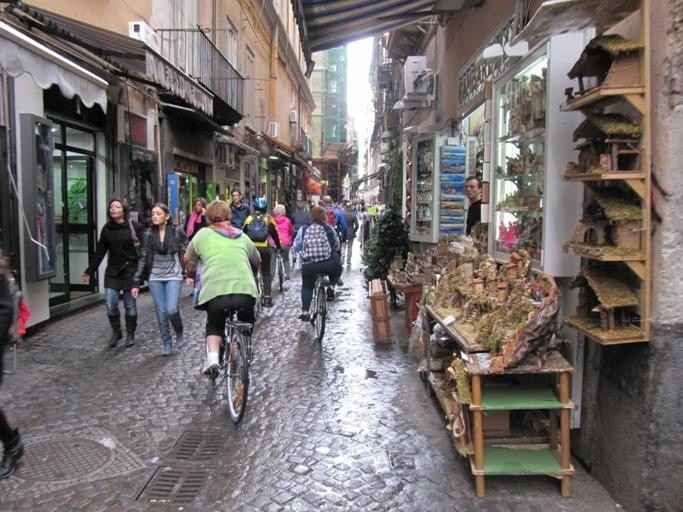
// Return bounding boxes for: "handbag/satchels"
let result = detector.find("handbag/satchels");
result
[128,218,146,257]
[175,226,187,266]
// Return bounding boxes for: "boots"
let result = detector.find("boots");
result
[125,312,138,347]
[0,427,25,479]
[169,311,185,348]
[108,312,122,347]
[157,311,173,356]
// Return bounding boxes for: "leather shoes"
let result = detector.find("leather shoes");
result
[327,287,334,295]
[299,314,310,321]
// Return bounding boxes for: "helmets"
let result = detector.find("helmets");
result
[253,197,268,208]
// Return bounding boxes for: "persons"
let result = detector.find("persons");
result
[319,195,347,240]
[464,176,482,235]
[243,198,281,307]
[307,199,314,209]
[343,201,359,266]
[291,199,310,265]
[80,199,145,347]
[131,203,194,356]
[317,199,324,206]
[332,197,343,211]
[229,189,251,228]
[293,206,342,322]
[269,204,294,281]
[183,197,209,243]
[185,200,262,375]
[0,249,31,479]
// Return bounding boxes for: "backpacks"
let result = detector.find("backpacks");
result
[301,219,332,262]
[245,212,269,242]
[1,267,28,347]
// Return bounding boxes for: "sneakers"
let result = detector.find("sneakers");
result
[201,360,220,373]
[264,297,272,307]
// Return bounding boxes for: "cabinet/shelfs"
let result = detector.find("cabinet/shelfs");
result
[508,1,654,347]
[409,132,469,243]
[386,271,424,329]
[424,302,578,499]
[488,30,594,279]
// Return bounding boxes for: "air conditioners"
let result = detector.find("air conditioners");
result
[129,21,162,55]
[290,112,298,122]
[404,56,437,102]
[269,122,278,138]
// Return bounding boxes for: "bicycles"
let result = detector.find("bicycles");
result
[209,303,253,423]
[254,244,277,320]
[309,272,331,339]
[276,252,284,292]
